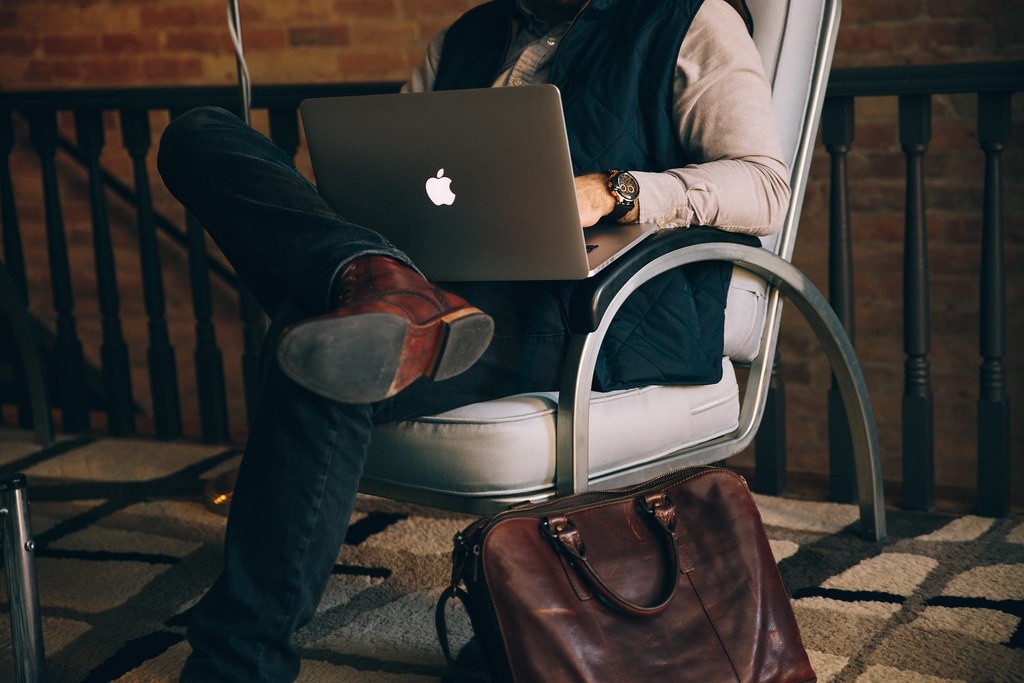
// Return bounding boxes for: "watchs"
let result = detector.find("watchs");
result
[596,168,640,224]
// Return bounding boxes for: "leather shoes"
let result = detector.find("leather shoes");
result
[273,253,496,404]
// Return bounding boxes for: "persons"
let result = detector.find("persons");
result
[156,0,793,683]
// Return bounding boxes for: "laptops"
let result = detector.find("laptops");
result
[298,84,658,281]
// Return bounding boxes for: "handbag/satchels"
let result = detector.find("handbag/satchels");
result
[435,464,816,683]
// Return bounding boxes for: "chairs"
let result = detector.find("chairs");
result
[355,0,887,553]
